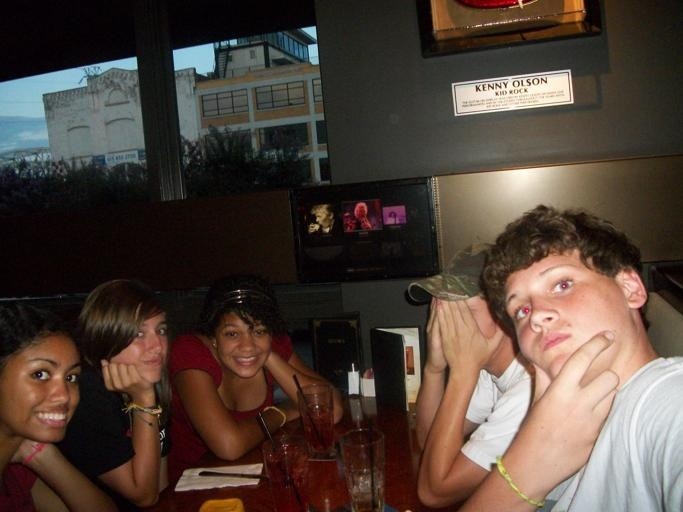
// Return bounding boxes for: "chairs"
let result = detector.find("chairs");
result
[645,261,682,358]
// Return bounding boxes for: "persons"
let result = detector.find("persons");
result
[402,242,535,509]
[168,271,346,480]
[353,202,373,230]
[386,211,400,224]
[58,277,171,507]
[453,202,682,512]
[308,204,336,235]
[344,211,357,230]
[0,301,120,511]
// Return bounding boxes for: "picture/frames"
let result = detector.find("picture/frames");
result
[414,0,601,58]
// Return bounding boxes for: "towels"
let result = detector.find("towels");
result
[174,464,265,491]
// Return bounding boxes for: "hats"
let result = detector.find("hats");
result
[407,241,497,303]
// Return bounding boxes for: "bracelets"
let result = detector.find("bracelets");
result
[494,453,548,509]
[121,400,164,425]
[261,403,289,429]
[21,441,46,467]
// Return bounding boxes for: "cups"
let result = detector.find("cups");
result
[360,378,376,397]
[363,398,377,415]
[296,384,335,459]
[340,428,385,511]
[262,439,310,512]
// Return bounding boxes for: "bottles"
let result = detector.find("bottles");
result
[349,399,364,432]
[347,363,360,395]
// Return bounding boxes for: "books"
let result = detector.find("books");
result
[307,310,362,391]
[370,325,422,414]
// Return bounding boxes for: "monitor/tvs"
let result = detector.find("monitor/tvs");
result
[290,176,440,284]
